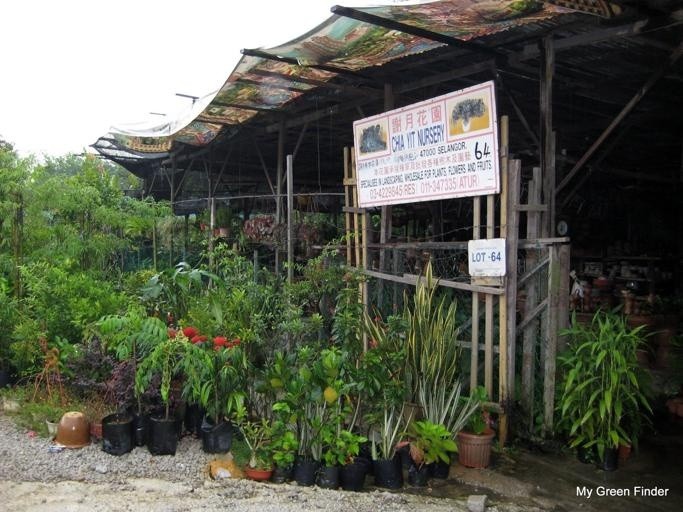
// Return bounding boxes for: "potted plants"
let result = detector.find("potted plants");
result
[102,231,669,491]
